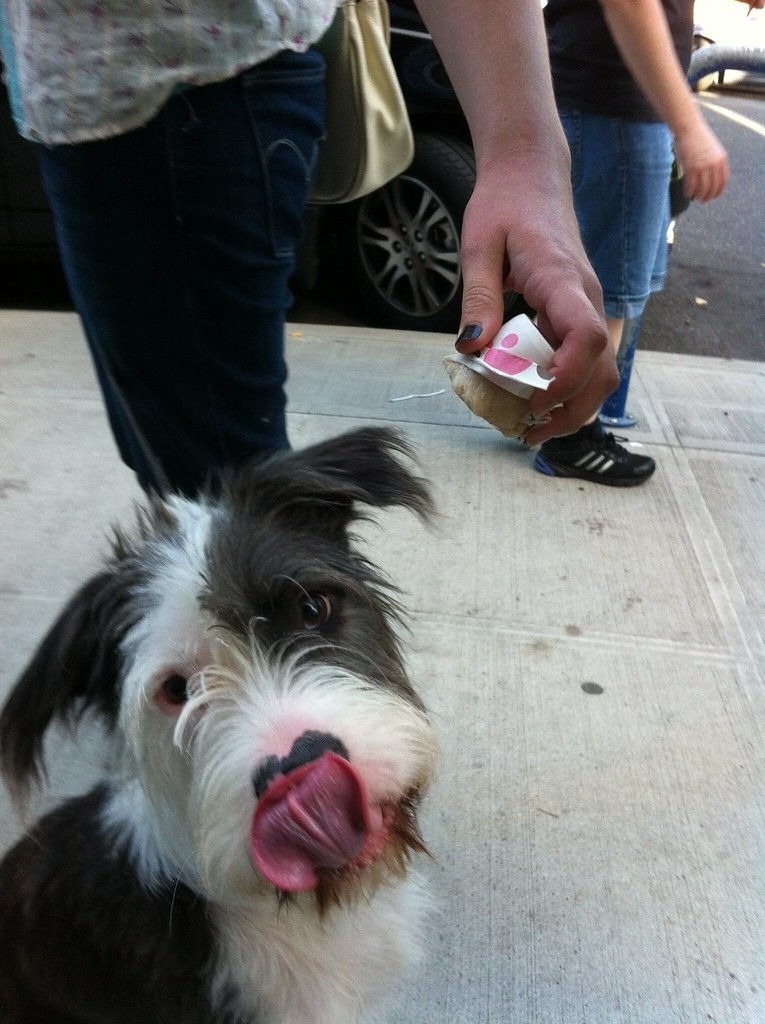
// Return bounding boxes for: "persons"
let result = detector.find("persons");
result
[0,0,619,518]
[533,0,727,486]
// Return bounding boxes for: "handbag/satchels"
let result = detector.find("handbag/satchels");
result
[305,0,416,204]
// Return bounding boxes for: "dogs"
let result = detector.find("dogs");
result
[1,421,456,1024]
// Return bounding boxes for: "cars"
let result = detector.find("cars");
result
[0,1,513,336]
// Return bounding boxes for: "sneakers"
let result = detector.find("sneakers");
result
[534,428,655,488]
